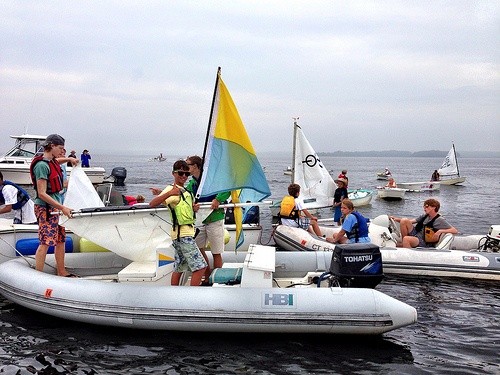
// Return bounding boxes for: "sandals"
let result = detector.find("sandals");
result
[65,273,78,278]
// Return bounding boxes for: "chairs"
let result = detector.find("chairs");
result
[436,232,454,249]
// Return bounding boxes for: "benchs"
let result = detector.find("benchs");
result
[119,240,171,282]
[210,243,275,287]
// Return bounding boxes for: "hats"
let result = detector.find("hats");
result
[334,178,348,187]
[40,134,65,146]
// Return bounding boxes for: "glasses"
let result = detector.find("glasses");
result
[423,204,430,208]
[172,169,191,177]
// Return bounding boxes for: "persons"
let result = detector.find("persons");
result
[429,170,439,188]
[149,160,209,286]
[326,201,372,245]
[0,171,38,225]
[31,134,79,278]
[186,156,232,286]
[59,148,92,207]
[389,199,458,248]
[385,178,397,188]
[280,184,326,238]
[384,168,392,176]
[332,170,349,226]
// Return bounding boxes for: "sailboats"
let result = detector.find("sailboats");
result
[284,117,374,209]
[438,143,467,185]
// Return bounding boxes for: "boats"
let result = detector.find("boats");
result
[376,172,441,198]
[273,215,500,280]
[0,66,418,334]
